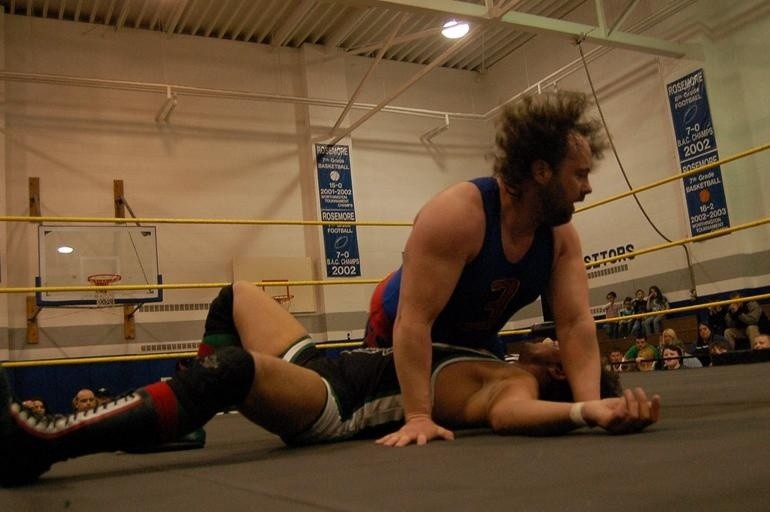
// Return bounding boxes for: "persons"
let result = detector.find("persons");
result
[604,285,770,371]
[22,397,48,415]
[362,92,614,448]
[0,281,661,489]
[72,387,113,412]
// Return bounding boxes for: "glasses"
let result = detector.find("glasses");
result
[96,391,110,398]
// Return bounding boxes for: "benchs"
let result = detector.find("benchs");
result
[591,313,701,362]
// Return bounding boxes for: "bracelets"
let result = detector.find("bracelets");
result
[570,402,588,427]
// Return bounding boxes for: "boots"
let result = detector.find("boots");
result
[0,367,170,489]
[121,341,216,453]
